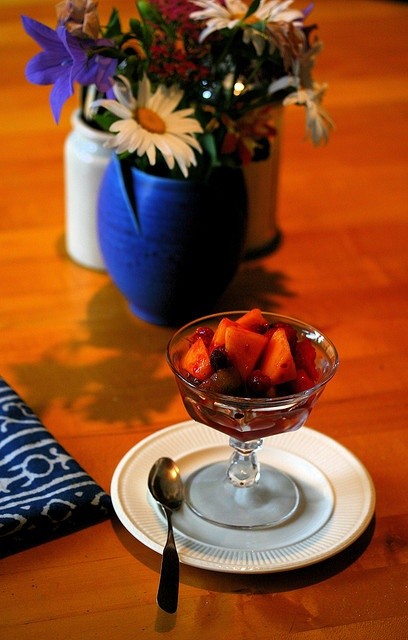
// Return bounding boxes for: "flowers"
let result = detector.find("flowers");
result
[19,0,335,181]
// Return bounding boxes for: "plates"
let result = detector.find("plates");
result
[111,420,376,575]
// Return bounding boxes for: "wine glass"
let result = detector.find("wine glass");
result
[167,310,340,529]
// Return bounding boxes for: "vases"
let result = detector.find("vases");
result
[99,147,249,326]
[246,128,284,257]
[62,110,125,271]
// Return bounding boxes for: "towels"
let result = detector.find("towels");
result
[0,375,111,537]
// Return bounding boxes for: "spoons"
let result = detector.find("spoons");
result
[147,456,187,614]
